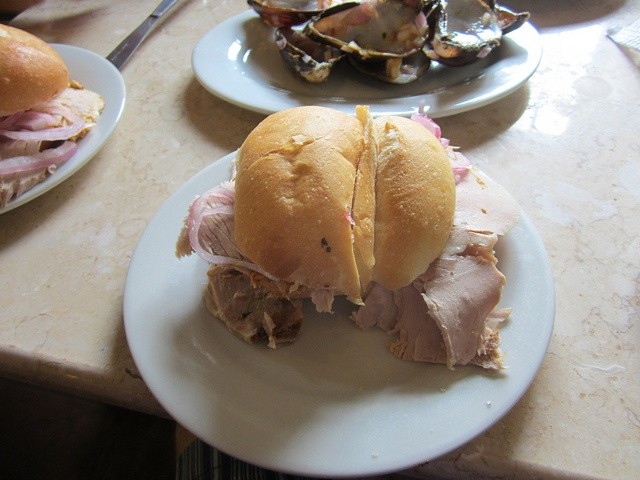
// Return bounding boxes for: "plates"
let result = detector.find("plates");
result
[124,148,558,478]
[0,43,127,215]
[191,7,544,118]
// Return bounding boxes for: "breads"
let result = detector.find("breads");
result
[0,23,69,117]
[235,104,456,307]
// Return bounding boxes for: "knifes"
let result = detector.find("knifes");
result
[106,0,187,72]
